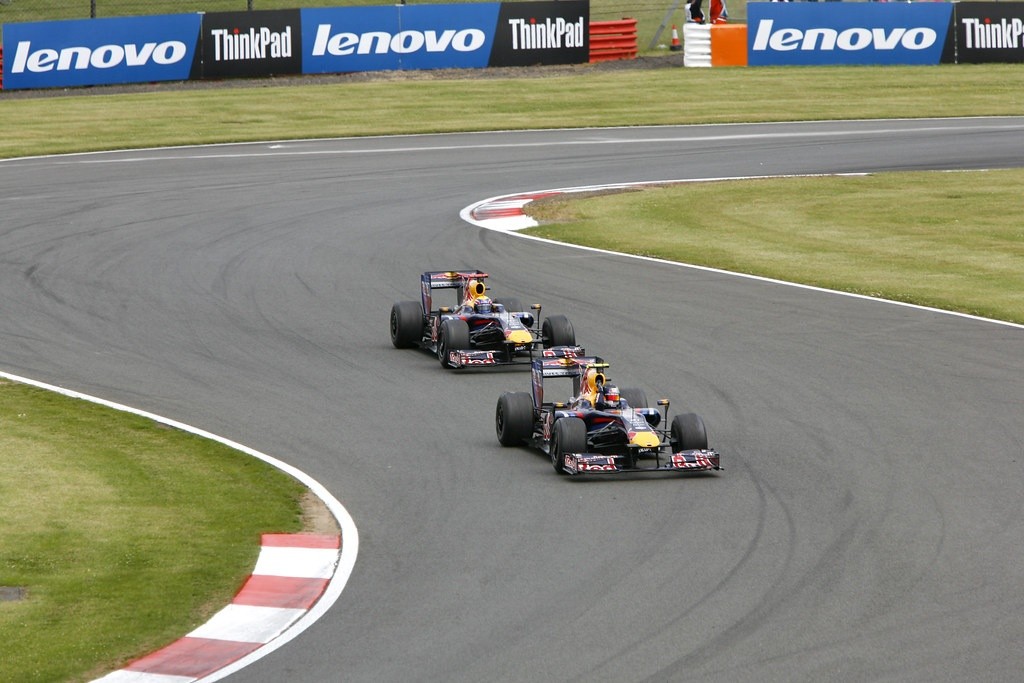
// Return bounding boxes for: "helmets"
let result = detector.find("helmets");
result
[602,383,621,405]
[475,296,492,314]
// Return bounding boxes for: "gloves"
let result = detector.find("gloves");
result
[596,379,603,392]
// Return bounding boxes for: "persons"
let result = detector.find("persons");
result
[596,383,621,409]
[685,0,706,22]
[474,296,494,314]
[708,0,729,24]
[689,0,705,23]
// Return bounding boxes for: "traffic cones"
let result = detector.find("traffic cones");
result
[669,24,682,50]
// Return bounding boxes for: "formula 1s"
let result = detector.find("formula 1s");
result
[389,268,576,369]
[492,345,725,478]
